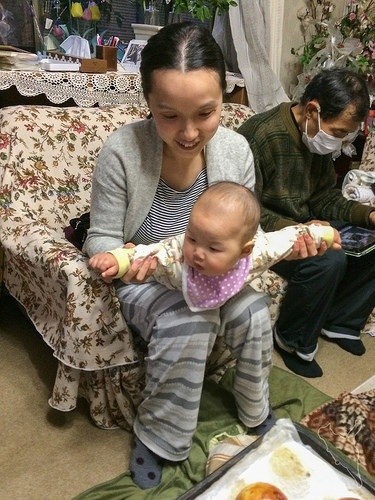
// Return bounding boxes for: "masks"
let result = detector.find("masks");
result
[302,110,342,155]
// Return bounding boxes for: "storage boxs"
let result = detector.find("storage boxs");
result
[176,421,375,500]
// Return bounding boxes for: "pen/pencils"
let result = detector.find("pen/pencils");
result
[97,34,119,47]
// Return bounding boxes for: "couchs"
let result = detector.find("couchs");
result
[0,102,289,430]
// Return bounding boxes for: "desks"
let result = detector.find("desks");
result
[0,69,245,107]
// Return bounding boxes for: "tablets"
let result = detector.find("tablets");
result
[336,223,375,258]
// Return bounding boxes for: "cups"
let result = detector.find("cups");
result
[96,45,118,71]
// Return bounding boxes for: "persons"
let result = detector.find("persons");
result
[236,69,375,379]
[84,24,343,490]
[88,180,340,312]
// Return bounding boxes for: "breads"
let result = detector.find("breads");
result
[234,481,289,500]
